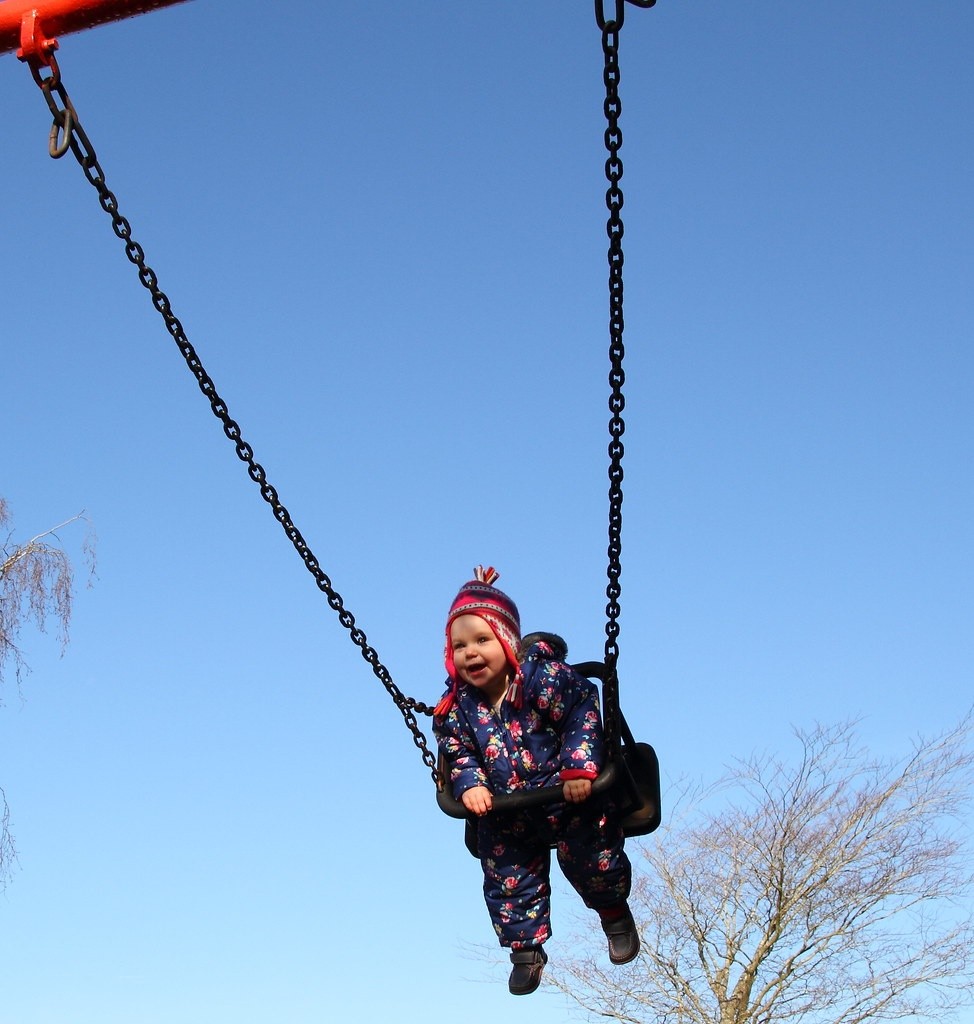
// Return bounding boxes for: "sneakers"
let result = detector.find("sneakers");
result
[509,944,548,995]
[601,914,640,965]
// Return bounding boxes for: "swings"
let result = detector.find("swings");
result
[17,1,662,861]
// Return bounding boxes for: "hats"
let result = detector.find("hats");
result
[434,566,525,718]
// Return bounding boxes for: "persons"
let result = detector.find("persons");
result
[432,565,641,995]
